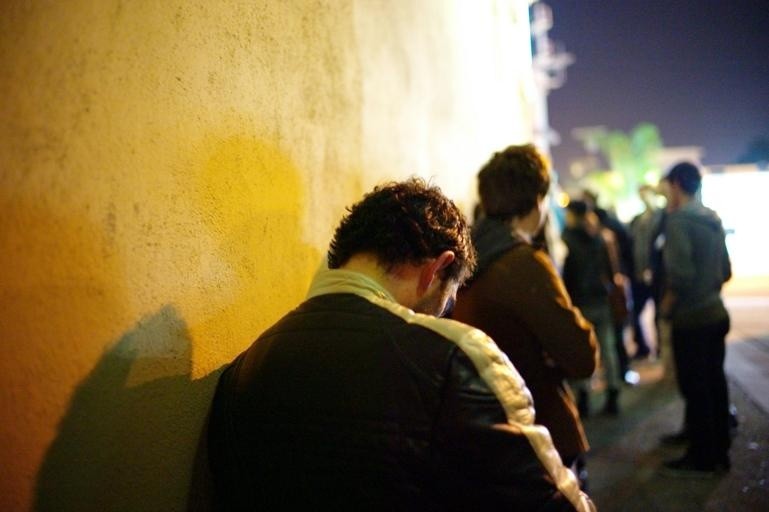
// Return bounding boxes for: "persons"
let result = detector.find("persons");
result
[183,176,601,512]
[654,161,729,479]
[435,140,600,494]
[474,178,739,430]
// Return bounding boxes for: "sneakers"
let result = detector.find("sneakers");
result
[579,347,738,473]
